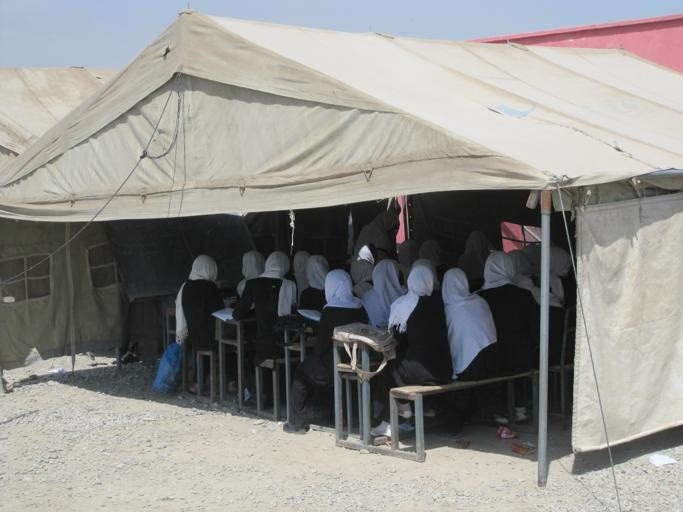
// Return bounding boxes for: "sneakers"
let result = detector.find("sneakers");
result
[397,401,412,418]
[370,420,392,438]
[284,422,306,434]
[412,407,437,418]
[486,396,534,426]
[186,375,281,408]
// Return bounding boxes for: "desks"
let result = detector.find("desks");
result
[165,307,198,399]
[276,320,332,431]
[331,328,398,442]
[215,315,259,409]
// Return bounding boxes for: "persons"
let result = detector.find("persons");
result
[167,211,577,446]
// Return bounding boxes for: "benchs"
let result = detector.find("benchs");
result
[388,368,540,452]
[253,356,302,420]
[194,349,236,405]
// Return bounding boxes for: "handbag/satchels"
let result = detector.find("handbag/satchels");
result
[332,321,398,380]
